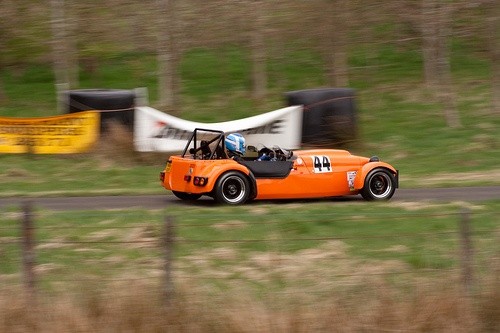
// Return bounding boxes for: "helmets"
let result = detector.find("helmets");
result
[224,133,246,155]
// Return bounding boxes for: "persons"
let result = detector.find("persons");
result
[225,132,276,161]
